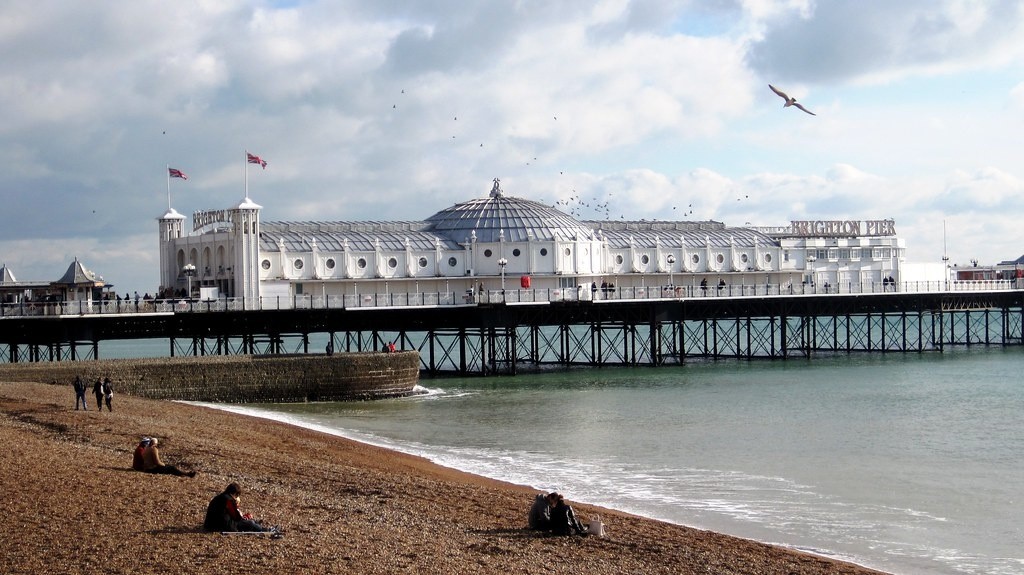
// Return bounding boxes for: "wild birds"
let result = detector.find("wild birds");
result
[392,90,750,225]
[768,84,816,115]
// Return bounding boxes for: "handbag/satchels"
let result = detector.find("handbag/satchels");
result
[106,390,113,399]
[100,385,105,395]
[589,514,605,536]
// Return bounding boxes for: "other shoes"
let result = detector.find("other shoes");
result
[190,471,197,477]
[99,408,101,411]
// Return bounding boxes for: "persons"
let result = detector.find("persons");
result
[203,483,274,533]
[102,377,114,412]
[326,341,332,356]
[73,375,87,411]
[102,287,188,305]
[133,437,196,478]
[469,278,727,297]
[92,377,104,411]
[528,492,588,536]
[382,341,396,354]
[883,275,895,285]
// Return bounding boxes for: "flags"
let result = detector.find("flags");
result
[169,168,188,180]
[247,153,267,170]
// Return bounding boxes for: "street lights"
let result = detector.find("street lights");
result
[940,254,949,291]
[497,257,508,305]
[667,256,676,297]
[181,263,196,313]
[805,256,817,295]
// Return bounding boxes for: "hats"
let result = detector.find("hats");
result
[141,437,150,442]
[104,378,111,383]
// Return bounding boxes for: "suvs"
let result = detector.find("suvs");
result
[35,294,63,312]
